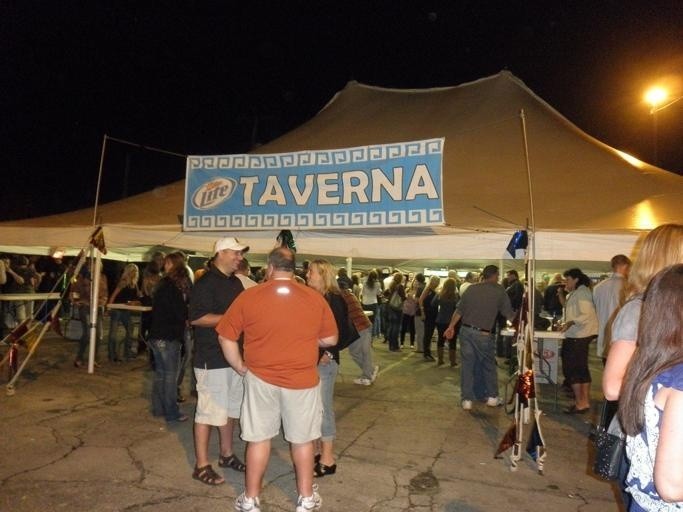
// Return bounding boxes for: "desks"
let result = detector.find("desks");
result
[498,327,567,414]
[107,303,153,365]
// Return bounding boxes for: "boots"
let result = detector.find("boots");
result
[447,346,458,368]
[436,343,445,367]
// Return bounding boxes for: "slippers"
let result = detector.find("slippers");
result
[564,405,591,414]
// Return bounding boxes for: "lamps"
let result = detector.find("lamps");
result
[506,230,528,259]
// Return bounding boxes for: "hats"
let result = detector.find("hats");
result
[211,235,251,259]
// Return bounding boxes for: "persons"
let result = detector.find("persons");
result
[383,272,406,352]
[305,257,346,479]
[400,281,416,351]
[592,254,632,369]
[617,262,682,512]
[434,278,460,368]
[214,247,338,512]
[417,275,441,363]
[163,253,194,403]
[351,274,361,298]
[556,267,599,416]
[544,274,568,318]
[174,250,193,285]
[534,270,552,328]
[380,268,401,294]
[411,273,426,354]
[598,223,683,431]
[68,257,111,368]
[361,270,381,349]
[442,264,515,410]
[194,255,214,283]
[188,237,249,485]
[108,261,141,364]
[233,257,259,291]
[505,268,525,358]
[457,271,476,299]
[336,266,352,289]
[372,268,385,340]
[298,259,310,282]
[494,277,508,359]
[0,253,75,330]
[146,264,191,423]
[247,263,256,282]
[140,259,162,299]
[152,251,166,276]
[447,268,462,289]
[338,279,380,387]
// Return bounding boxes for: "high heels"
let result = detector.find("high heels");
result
[309,463,338,478]
[424,354,436,363]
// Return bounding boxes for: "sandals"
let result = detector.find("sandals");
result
[94,360,102,369]
[186,463,226,487]
[75,360,86,370]
[169,414,188,423]
[178,395,187,405]
[216,453,247,474]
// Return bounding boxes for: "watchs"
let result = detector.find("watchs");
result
[322,351,336,361]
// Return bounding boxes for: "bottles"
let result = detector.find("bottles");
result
[61,270,69,297]
[552,312,557,331]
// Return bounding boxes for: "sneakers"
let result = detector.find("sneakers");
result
[293,490,324,512]
[460,396,474,411]
[401,343,405,350]
[408,345,415,349]
[484,394,505,408]
[368,362,381,382]
[350,377,372,387]
[229,492,261,512]
